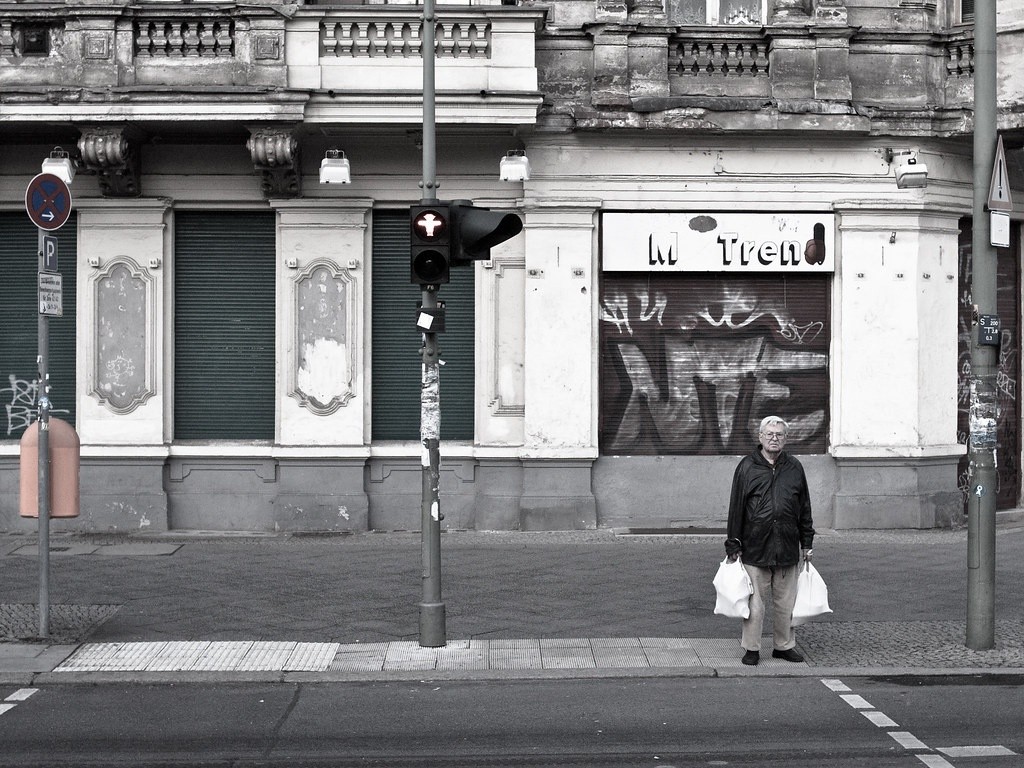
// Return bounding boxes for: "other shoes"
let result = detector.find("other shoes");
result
[741,649,759,665]
[772,648,804,663]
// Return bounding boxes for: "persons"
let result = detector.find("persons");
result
[723,414,816,665]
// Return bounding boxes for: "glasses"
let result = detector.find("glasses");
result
[760,432,785,440]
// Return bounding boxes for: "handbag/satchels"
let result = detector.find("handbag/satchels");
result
[713,554,754,619]
[790,550,832,628]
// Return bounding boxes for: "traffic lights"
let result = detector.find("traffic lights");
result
[409,206,523,284]
